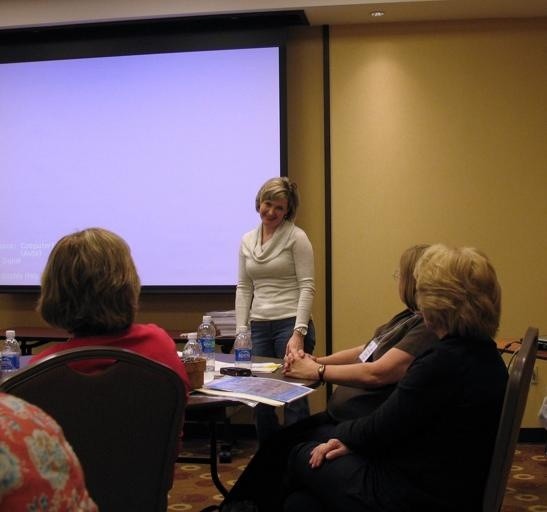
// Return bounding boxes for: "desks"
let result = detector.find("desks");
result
[175,353,322,498]
[0,326,236,354]
[497,344,547,360]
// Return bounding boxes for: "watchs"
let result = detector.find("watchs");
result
[296,328,307,336]
[318,364,325,383]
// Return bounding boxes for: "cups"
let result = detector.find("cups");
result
[179,357,205,388]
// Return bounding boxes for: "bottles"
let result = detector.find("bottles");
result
[234,326,253,364]
[0,330,20,375]
[184,315,215,373]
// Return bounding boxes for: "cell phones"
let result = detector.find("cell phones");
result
[220,366,252,376]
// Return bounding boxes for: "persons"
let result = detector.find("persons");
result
[280,242,510,512]
[217,243,440,512]
[234,176,316,441]
[0,229,191,455]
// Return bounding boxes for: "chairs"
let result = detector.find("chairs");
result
[483,327,539,512]
[0,346,187,512]
